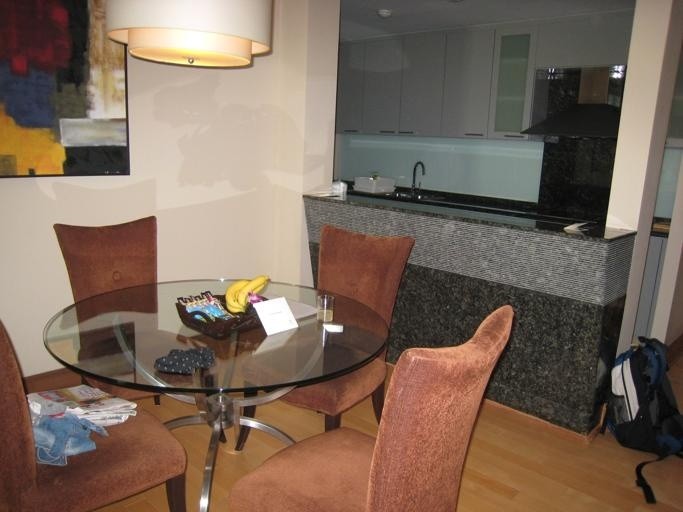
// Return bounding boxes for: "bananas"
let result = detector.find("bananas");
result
[224,274,271,314]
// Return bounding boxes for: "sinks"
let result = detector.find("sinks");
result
[374,189,446,201]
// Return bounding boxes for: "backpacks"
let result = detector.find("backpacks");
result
[609,336,683,456]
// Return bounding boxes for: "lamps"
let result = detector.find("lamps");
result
[106,1,273,69]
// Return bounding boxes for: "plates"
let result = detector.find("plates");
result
[175,294,268,340]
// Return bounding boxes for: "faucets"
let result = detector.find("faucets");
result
[411,162,425,195]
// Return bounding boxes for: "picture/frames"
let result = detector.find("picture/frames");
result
[0,0,131,179]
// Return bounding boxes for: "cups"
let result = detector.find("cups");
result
[317,295,335,322]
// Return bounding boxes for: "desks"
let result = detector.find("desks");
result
[43,278,390,512]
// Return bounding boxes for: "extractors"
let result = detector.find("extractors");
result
[519,67,621,139]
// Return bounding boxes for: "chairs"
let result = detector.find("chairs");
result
[235,224,415,451]
[232,304,515,512]
[53,215,228,443]
[0,320,188,512]
